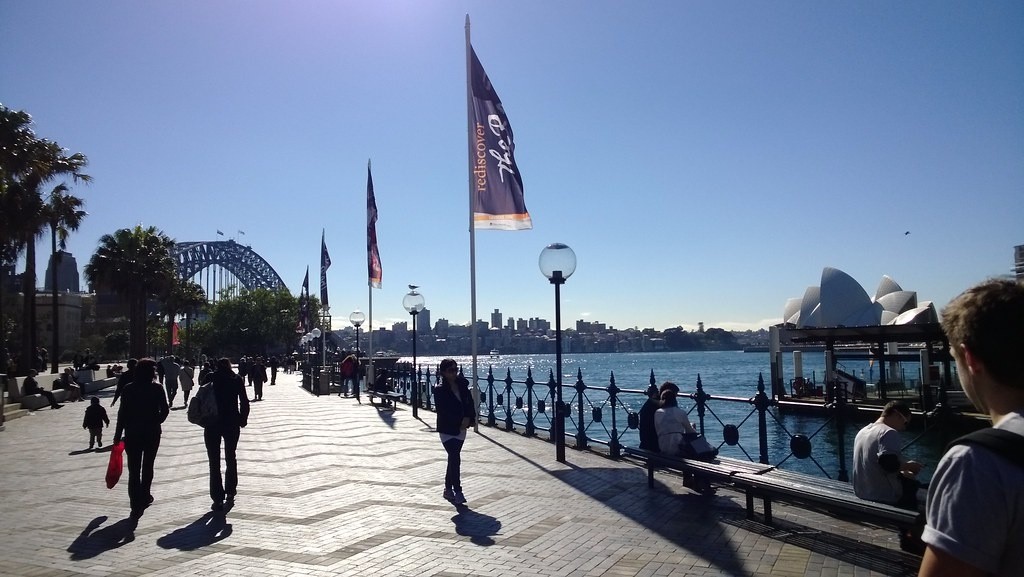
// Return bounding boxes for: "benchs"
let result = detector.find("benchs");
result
[874,381,912,396]
[94,368,123,388]
[362,381,406,411]
[729,467,925,558]
[9,370,104,411]
[624,446,776,519]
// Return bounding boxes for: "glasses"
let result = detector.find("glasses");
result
[447,368,458,373]
[893,408,909,427]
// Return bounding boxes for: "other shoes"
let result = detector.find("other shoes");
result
[211,503,223,510]
[226,500,234,507]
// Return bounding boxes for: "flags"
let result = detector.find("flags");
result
[303,270,309,295]
[240,231,245,235]
[217,230,223,235]
[366,167,382,288]
[321,236,331,306]
[469,44,532,232]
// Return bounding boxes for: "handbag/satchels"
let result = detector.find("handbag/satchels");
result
[106,441,124,488]
[680,432,718,457]
[188,379,214,428]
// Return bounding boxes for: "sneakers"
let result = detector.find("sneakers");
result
[443,488,454,500]
[455,493,466,504]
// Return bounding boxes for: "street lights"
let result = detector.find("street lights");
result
[299,327,322,369]
[349,311,366,399]
[402,290,425,417]
[537,242,579,462]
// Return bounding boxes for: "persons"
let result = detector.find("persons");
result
[434,358,476,503]
[917,278,1024,577]
[201,358,250,509]
[338,354,395,404]
[36,347,100,402]
[238,355,279,400]
[638,382,718,495]
[113,359,170,510]
[156,354,218,407]
[106,365,124,378]
[83,396,110,448]
[280,354,295,374]
[23,369,65,409]
[853,400,927,551]
